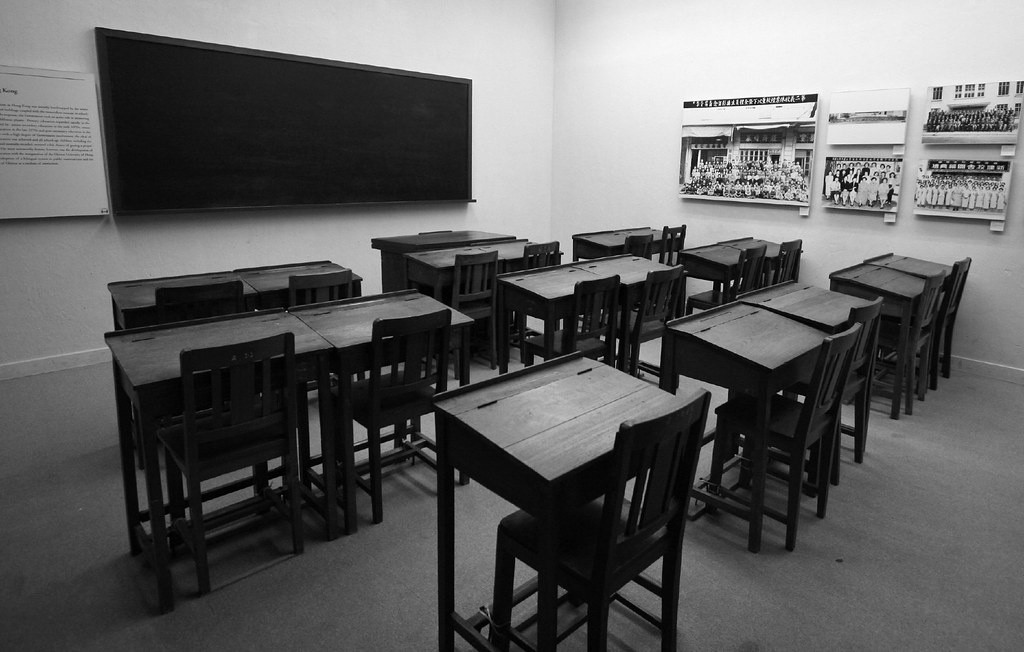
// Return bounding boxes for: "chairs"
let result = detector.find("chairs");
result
[451,249,499,380]
[155,280,245,325]
[660,224,686,265]
[524,272,620,367]
[784,296,885,484]
[599,263,683,378]
[512,241,560,342]
[772,239,802,285]
[686,243,767,316]
[489,389,711,650]
[158,331,304,596]
[886,256,972,386]
[331,308,451,524]
[870,269,946,414]
[706,323,865,551]
[624,234,653,260]
[289,267,364,392]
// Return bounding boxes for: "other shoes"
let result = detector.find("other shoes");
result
[826,197,830,200]
[917,204,1004,213]
[834,201,892,209]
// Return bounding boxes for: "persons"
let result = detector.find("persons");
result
[679,106,1016,213]
[825,171,834,200]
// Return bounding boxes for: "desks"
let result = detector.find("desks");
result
[103,228,958,652]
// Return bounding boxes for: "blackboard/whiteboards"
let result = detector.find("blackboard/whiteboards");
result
[95,27,477,216]
